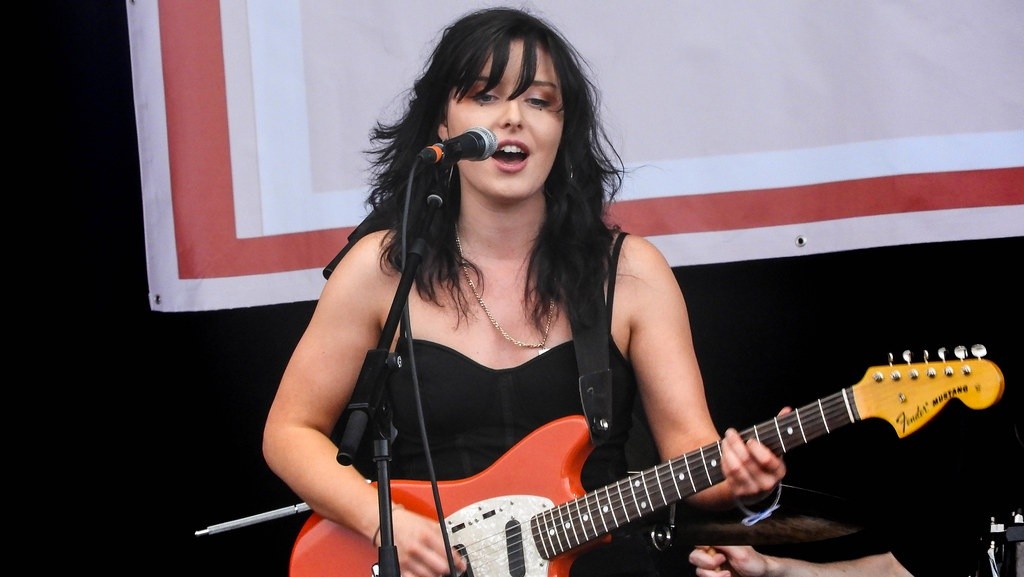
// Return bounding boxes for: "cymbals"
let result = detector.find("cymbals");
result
[624,471,871,547]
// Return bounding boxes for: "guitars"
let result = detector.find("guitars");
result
[287,338,1007,577]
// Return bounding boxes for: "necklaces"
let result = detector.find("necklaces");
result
[453,217,555,348]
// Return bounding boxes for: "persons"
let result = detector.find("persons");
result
[686,471,1024,577]
[260,8,794,577]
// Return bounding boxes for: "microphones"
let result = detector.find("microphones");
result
[421,127,499,162]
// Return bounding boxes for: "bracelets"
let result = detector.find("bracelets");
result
[728,477,784,526]
[371,503,406,549]
